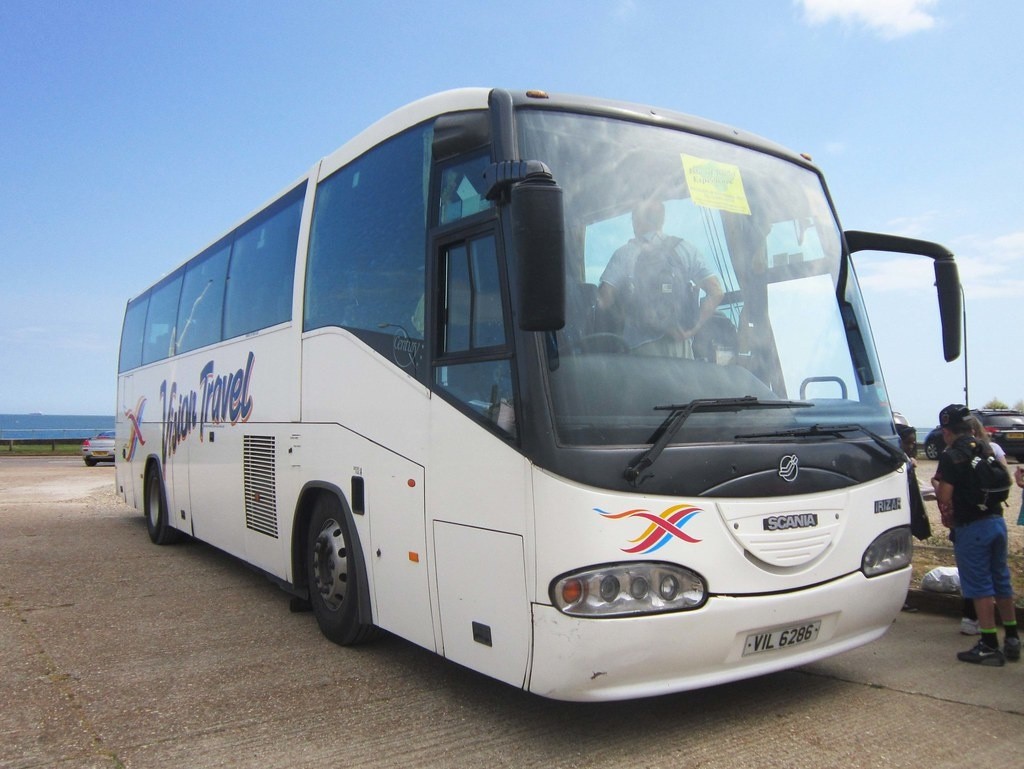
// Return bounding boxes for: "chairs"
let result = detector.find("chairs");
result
[570,282,739,363]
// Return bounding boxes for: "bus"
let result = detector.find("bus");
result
[112,86,962,707]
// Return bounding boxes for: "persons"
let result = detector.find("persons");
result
[930,404,1021,667]
[594,193,723,367]
[892,422,917,614]
[1014,466,1024,527]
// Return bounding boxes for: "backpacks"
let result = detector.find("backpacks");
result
[628,235,699,334]
[953,444,1012,507]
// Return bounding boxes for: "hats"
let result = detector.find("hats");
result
[932,404,974,431]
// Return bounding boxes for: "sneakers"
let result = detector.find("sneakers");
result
[957,641,1005,667]
[1003,637,1021,660]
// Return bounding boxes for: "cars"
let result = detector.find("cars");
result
[924,408,1024,466]
[81,430,116,467]
[891,410,918,457]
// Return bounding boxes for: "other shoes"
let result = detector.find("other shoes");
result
[961,619,982,634]
